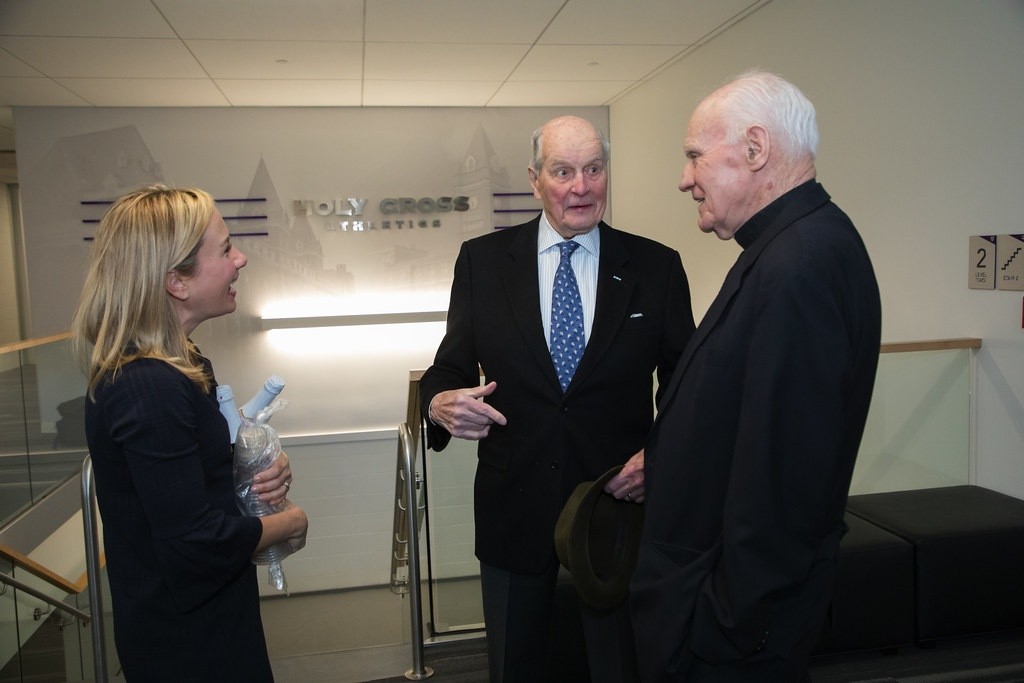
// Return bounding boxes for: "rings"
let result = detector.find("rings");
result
[627,493,633,501]
[282,481,290,491]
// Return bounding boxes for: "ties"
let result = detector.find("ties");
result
[549,241,586,394]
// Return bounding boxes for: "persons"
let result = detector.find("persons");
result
[417,115,698,683]
[603,69,882,683]
[69,182,307,683]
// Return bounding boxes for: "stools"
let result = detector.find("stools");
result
[830,481,1024,648]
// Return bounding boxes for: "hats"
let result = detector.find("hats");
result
[553,464,645,610]
